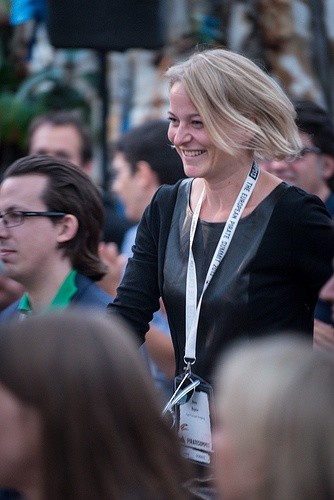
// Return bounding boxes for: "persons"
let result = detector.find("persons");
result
[208,330,334,500]
[257,97,333,360]
[28,110,94,174]
[102,49,334,500]
[96,117,189,436]
[0,155,165,421]
[0,305,197,500]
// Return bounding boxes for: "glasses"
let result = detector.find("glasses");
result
[0,211,65,229]
[160,373,200,430]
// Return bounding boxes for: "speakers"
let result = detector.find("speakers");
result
[46,0,164,53]
